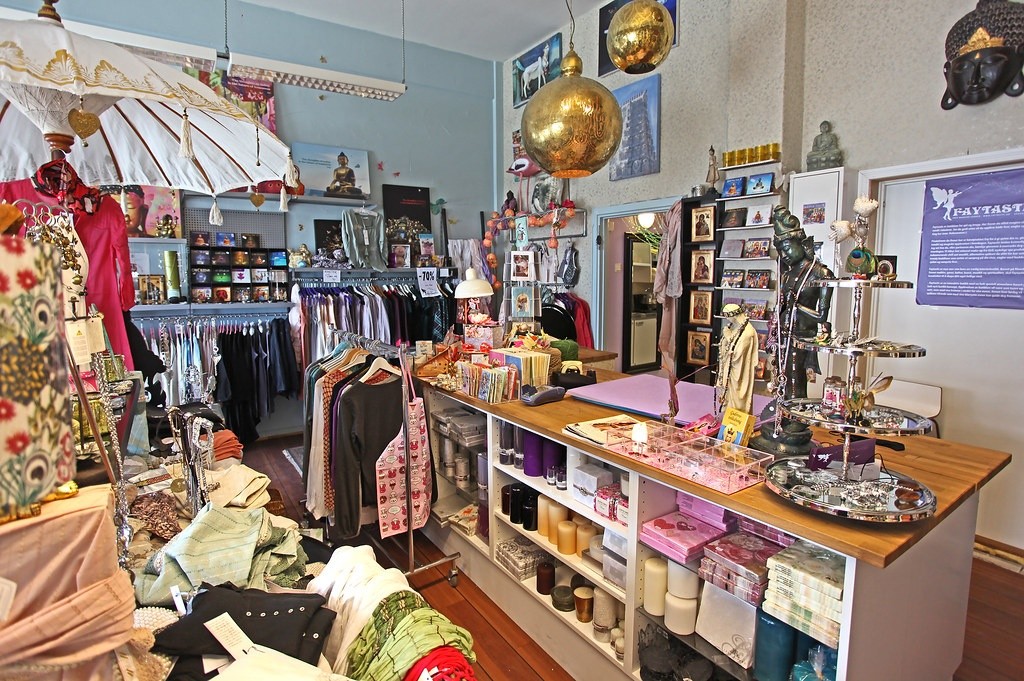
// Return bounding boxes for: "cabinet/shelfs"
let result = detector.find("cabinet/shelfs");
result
[710,158,780,382]
[428,387,1014,681]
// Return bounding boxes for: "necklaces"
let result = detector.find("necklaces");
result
[714,316,749,415]
[93,352,134,572]
[167,406,221,513]
[772,255,817,437]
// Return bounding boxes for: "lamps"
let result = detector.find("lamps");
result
[453,268,494,343]
[606,0,674,74]
[521,0,622,180]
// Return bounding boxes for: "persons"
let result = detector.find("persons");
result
[747,241,767,257]
[807,121,841,157]
[721,268,771,289]
[99,185,156,238]
[695,256,709,279]
[194,234,266,265]
[329,151,356,193]
[772,205,835,435]
[727,177,765,225]
[691,339,706,360]
[696,214,709,236]
[717,304,759,415]
[694,296,708,320]
[706,145,721,188]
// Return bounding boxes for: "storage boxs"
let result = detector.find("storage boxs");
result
[573,420,846,670]
[406,322,504,373]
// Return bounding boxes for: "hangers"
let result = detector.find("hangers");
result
[130,312,290,344]
[292,273,450,298]
[315,328,402,393]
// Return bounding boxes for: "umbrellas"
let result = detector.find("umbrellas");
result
[0,19,298,228]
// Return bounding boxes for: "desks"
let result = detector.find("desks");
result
[74,371,151,488]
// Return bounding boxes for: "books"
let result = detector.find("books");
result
[562,414,645,448]
[676,408,762,460]
[460,347,552,404]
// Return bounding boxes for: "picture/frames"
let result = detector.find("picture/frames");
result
[691,206,714,242]
[686,330,711,366]
[689,291,712,325]
[597,0,679,77]
[692,249,714,286]
